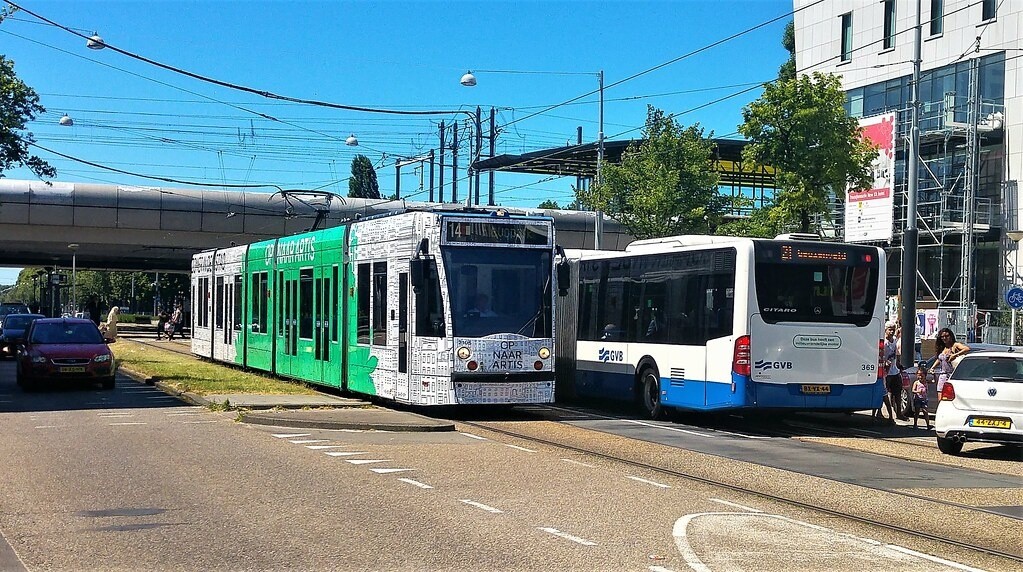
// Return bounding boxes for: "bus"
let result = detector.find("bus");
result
[555,211,888,422]
[195,214,571,408]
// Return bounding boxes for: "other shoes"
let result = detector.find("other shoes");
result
[889,418,895,425]
[913,426,918,432]
[877,414,884,419]
[897,414,909,421]
[927,425,933,430]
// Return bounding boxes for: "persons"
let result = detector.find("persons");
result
[872,387,896,425]
[108,306,119,337]
[885,322,909,421]
[930,327,970,402]
[169,304,186,341]
[157,306,175,340]
[894,319,902,368]
[647,312,665,337]
[911,368,934,430]
[601,324,615,339]
[915,324,924,367]
[464,295,497,318]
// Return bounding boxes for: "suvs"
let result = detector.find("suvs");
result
[0,302,31,328]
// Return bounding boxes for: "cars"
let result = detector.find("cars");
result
[889,342,1023,420]
[0,314,47,358]
[16,318,112,385]
[935,350,1023,454]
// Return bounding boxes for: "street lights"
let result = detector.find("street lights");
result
[36,270,47,312]
[459,68,606,252]
[68,244,78,319]
[31,274,39,313]
[43,266,54,317]
